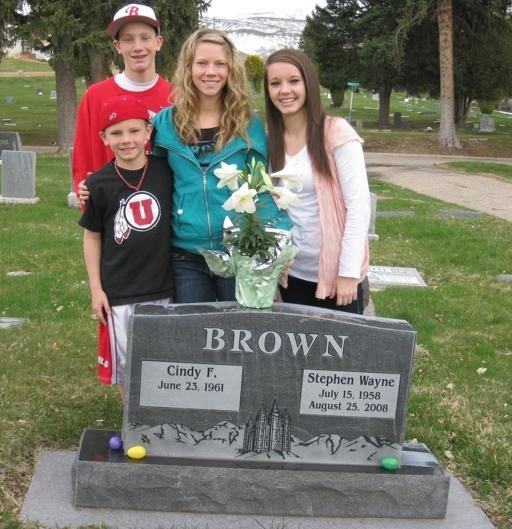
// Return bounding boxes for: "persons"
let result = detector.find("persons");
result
[262,47,373,317]
[75,94,175,403]
[73,3,177,219]
[78,27,293,302]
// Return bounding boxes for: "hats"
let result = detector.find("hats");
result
[108,3,160,40]
[100,95,157,140]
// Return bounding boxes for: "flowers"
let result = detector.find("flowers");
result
[214,154,304,267]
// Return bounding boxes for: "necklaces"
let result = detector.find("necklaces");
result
[114,155,148,193]
